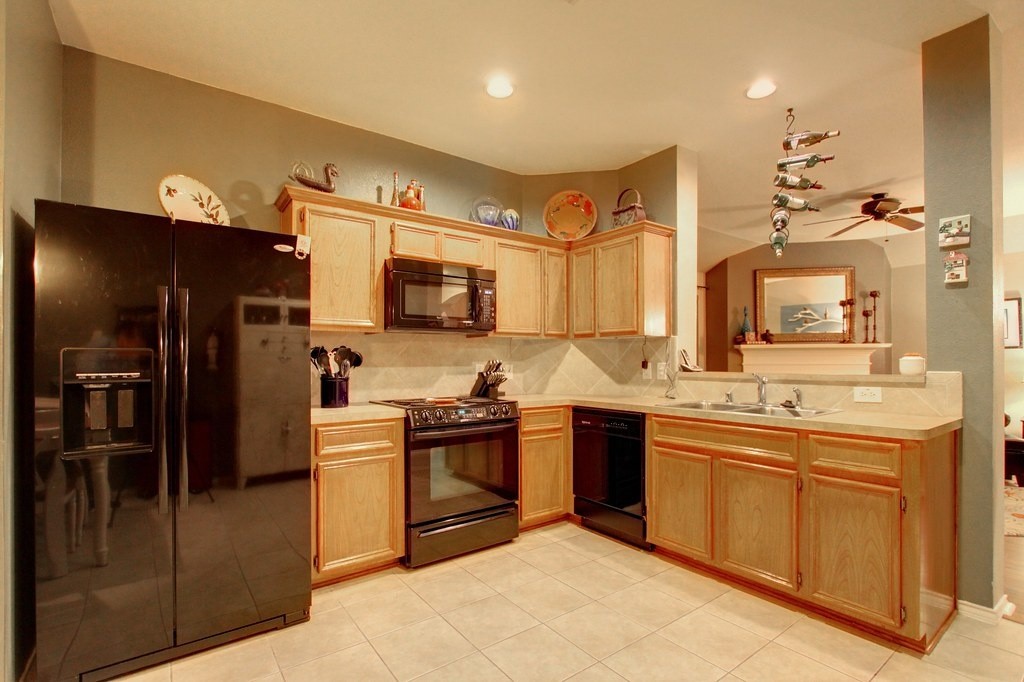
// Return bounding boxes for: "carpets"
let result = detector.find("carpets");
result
[1004,485,1024,536]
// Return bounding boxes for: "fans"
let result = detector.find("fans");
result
[803,191,924,238]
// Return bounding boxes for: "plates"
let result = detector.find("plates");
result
[544,189,598,241]
[159,174,231,227]
[469,193,504,226]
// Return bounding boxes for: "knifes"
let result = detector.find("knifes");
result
[473,358,507,386]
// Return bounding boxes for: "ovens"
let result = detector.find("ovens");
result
[383,257,497,336]
[400,426,520,568]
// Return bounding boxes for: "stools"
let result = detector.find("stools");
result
[35,467,79,552]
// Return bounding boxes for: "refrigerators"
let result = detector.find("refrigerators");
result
[23,197,312,682]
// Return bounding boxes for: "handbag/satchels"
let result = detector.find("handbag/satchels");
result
[611,188,646,229]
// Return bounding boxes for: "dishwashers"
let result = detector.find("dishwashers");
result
[571,406,645,545]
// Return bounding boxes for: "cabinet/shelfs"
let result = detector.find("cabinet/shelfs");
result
[236,295,310,489]
[495,236,637,334]
[807,432,902,627]
[300,205,378,332]
[648,416,803,592]
[391,221,484,267]
[521,408,565,523]
[315,421,397,573]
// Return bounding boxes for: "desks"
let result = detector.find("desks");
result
[34,399,113,580]
[1004,434,1024,487]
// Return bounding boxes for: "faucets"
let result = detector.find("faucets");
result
[751,371,768,405]
[724,391,733,402]
[792,386,802,408]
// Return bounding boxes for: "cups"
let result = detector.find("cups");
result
[477,205,500,225]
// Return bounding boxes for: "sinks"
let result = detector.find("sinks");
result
[738,407,844,418]
[655,401,752,411]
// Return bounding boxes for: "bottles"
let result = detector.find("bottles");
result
[769,228,787,258]
[390,169,426,213]
[770,208,792,231]
[776,152,834,170]
[783,130,841,150]
[501,208,520,231]
[770,194,822,212]
[741,304,752,338]
[772,175,824,190]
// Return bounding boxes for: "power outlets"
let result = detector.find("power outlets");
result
[642,362,651,380]
[853,386,882,403]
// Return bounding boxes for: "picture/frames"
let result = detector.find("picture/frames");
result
[754,267,855,341]
[1003,298,1023,349]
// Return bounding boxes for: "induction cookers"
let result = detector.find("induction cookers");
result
[373,396,521,429]
